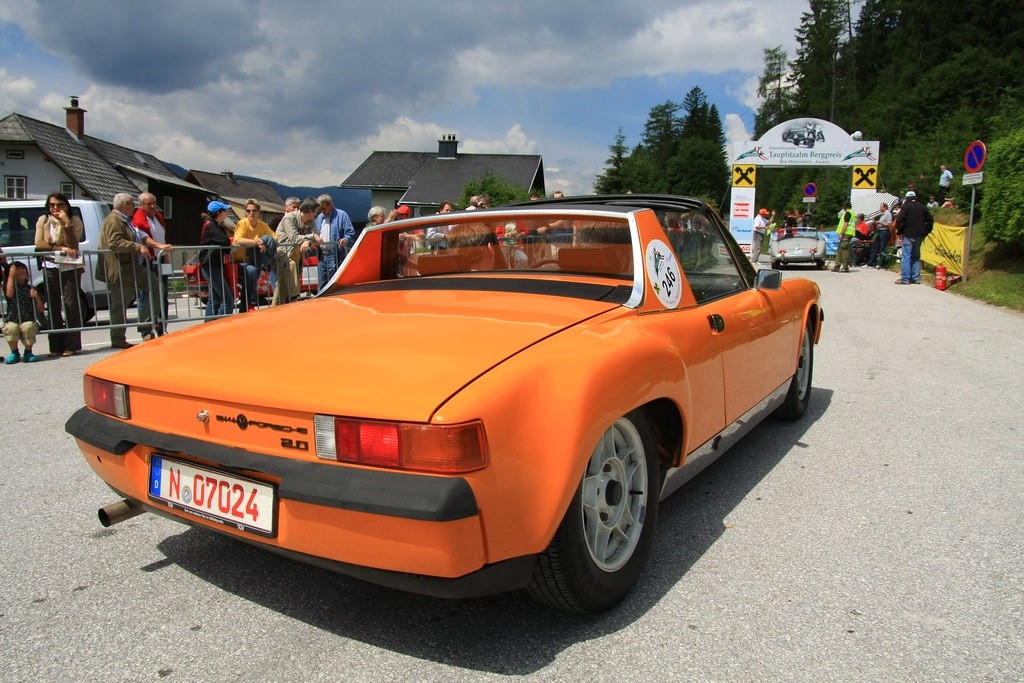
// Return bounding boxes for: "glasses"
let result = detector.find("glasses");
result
[49,203,66,208]
[127,202,135,207]
[246,209,257,213]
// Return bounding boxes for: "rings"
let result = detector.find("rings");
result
[260,243,261,244]
[306,246,309,249]
[258,242,259,243]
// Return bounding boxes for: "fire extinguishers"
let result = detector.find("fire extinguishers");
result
[934,261,946,291]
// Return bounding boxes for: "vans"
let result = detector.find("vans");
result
[0,198,139,330]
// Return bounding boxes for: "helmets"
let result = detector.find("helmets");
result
[842,201,851,208]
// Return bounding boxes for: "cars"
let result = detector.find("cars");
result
[52,189,832,626]
[181,235,351,305]
[769,225,827,269]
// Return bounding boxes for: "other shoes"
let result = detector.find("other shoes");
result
[831,268,840,271]
[48,352,62,356]
[63,350,75,356]
[895,278,909,285]
[111,343,137,349]
[875,265,881,268]
[841,268,849,272]
[750,262,761,266]
[861,265,870,267]
[143,325,162,341]
[23,352,40,361]
[5,353,20,364]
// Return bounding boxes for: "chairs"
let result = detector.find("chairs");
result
[557,248,621,275]
[2,222,28,247]
[413,255,472,276]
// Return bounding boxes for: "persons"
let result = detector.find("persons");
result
[655,209,713,262]
[231,198,278,313]
[496,199,529,269]
[99,193,149,348]
[577,220,632,272]
[766,207,808,237]
[198,201,234,323]
[271,194,355,307]
[465,195,489,210]
[849,213,879,267]
[0,247,45,364]
[132,193,173,341]
[750,209,775,266]
[35,193,85,357]
[862,203,892,268]
[530,193,554,234]
[895,191,935,285]
[365,206,399,227]
[447,223,495,270]
[396,205,425,273]
[426,201,454,251]
[831,201,855,272]
[893,165,956,217]
[549,191,570,228]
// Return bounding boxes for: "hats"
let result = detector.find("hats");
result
[396,205,411,214]
[899,191,904,193]
[759,208,769,215]
[207,200,231,213]
[905,190,916,197]
[907,183,914,188]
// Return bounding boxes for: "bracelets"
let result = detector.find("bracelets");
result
[864,240,866,242]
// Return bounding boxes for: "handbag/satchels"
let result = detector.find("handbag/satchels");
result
[32,248,43,271]
